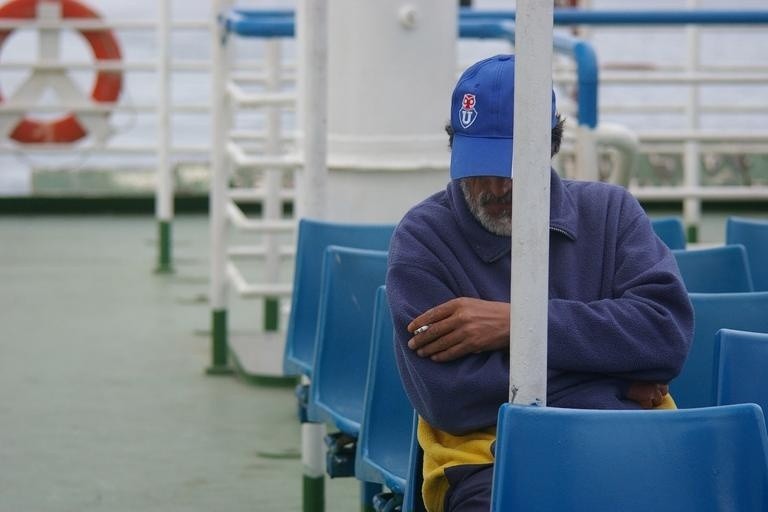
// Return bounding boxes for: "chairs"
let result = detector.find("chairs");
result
[280,215,768,510]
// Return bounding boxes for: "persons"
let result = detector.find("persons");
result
[386,54,696,510]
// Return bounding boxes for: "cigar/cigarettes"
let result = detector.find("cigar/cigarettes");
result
[414,324,432,336]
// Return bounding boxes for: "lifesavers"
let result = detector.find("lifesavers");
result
[1,0,123,143]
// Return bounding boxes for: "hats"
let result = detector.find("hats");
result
[448,52,559,181]
